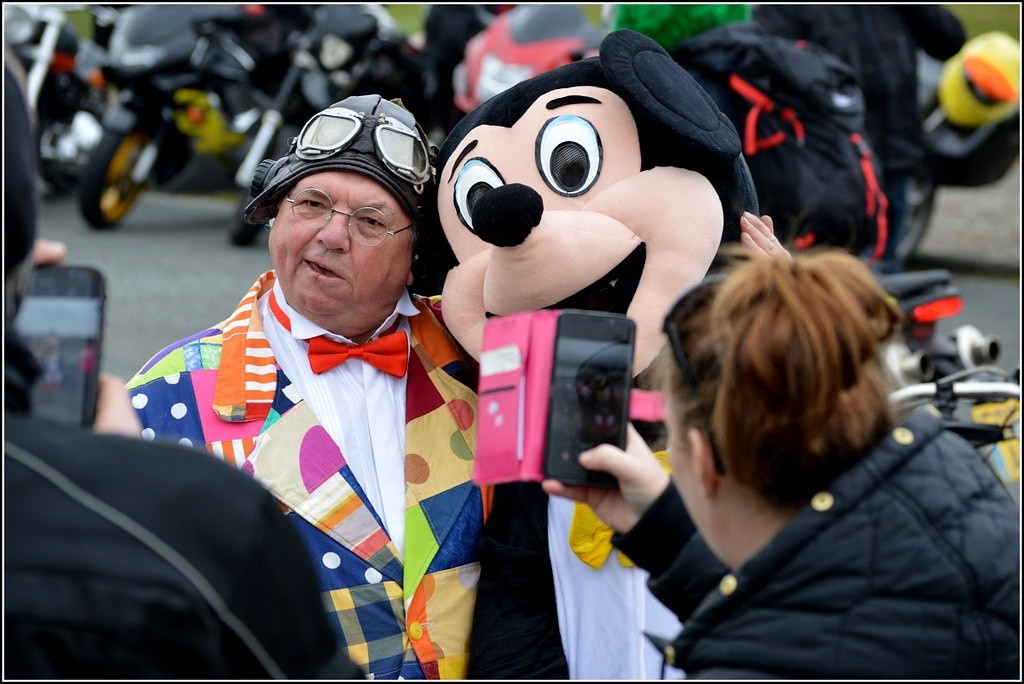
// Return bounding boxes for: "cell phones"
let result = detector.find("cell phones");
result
[542,311,636,490]
[12,263,106,430]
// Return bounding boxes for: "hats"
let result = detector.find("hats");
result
[4,63,35,281]
[244,94,440,294]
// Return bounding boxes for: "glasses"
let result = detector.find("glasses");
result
[285,107,435,184]
[287,188,414,247]
[662,276,726,476]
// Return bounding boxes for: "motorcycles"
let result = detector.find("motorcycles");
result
[78,5,303,233]
[229,5,436,246]
[875,272,1022,484]
[3,3,106,196]
[437,6,614,137]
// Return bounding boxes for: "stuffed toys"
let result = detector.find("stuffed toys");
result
[416,29,763,678]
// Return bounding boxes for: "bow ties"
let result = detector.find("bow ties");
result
[269,289,409,379]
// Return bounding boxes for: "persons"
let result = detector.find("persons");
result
[547,249,1019,682]
[126,92,793,684]
[4,63,345,681]
